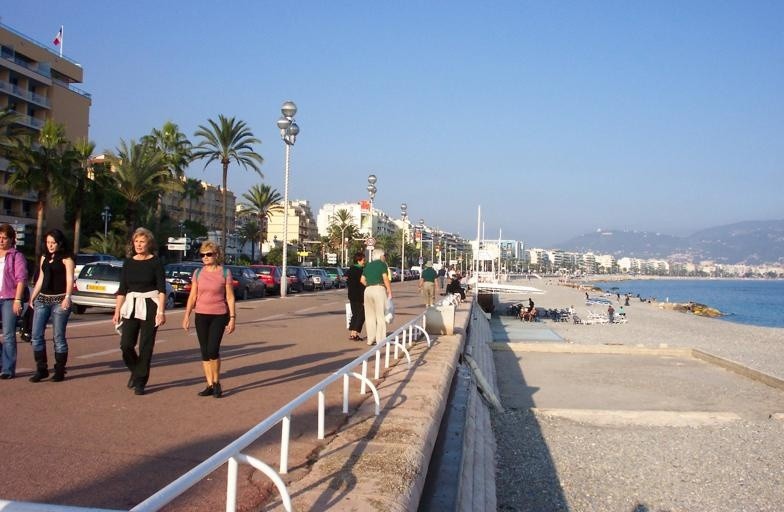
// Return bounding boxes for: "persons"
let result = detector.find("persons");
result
[26,228,75,382]
[360,248,392,346]
[110,227,167,395]
[418,258,470,306]
[180,239,235,397]
[0,224,28,379]
[506,289,656,324]
[342,253,366,340]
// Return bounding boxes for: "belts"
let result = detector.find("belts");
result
[36,295,66,304]
[365,284,386,287]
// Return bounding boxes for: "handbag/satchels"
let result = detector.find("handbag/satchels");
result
[345,303,352,329]
[383,296,395,324]
[21,286,30,303]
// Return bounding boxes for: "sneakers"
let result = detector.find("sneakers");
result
[197,385,212,396]
[0,373,15,379]
[133,379,146,395]
[212,381,221,397]
[426,304,434,309]
[126,372,135,389]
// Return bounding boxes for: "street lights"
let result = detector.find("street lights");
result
[275,99,299,297]
[99,205,113,239]
[334,220,354,267]
[365,174,376,263]
[430,234,473,279]
[399,201,407,281]
[417,218,424,278]
[176,219,188,261]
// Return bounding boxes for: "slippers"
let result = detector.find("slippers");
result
[347,335,363,341]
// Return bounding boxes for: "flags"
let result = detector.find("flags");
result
[52,28,61,46]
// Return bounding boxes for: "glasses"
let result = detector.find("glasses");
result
[199,252,215,258]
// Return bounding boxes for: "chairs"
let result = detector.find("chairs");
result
[511,297,628,326]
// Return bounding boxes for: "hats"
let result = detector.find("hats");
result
[424,261,434,267]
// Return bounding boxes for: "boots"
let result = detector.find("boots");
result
[50,351,68,382]
[28,349,49,384]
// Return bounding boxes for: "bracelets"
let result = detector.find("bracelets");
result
[64,294,72,298]
[229,316,235,319]
[14,298,23,303]
